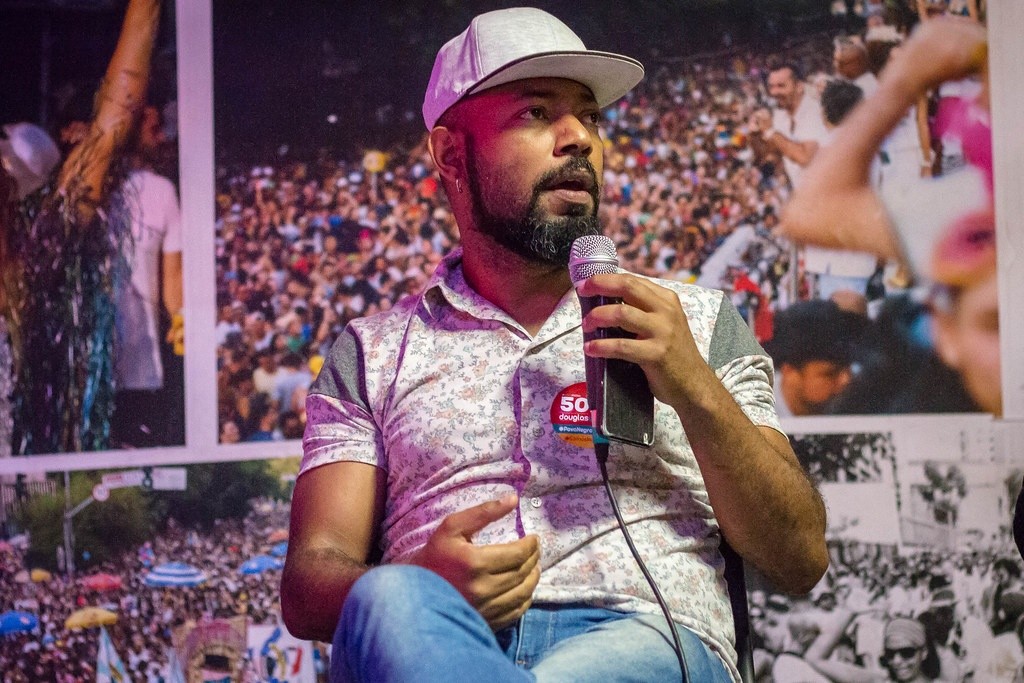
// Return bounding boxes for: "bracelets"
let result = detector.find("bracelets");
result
[762,127,775,139]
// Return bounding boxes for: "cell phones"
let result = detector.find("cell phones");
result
[597,292,656,447]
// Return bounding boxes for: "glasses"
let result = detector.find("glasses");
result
[885,647,925,661]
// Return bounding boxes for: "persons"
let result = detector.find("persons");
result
[0,0,1024,683]
[279,7,825,683]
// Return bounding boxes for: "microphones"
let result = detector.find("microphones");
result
[568,236,620,463]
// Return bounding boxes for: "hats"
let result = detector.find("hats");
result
[762,299,874,368]
[1,122,62,202]
[866,25,902,43]
[422,6,646,133]
